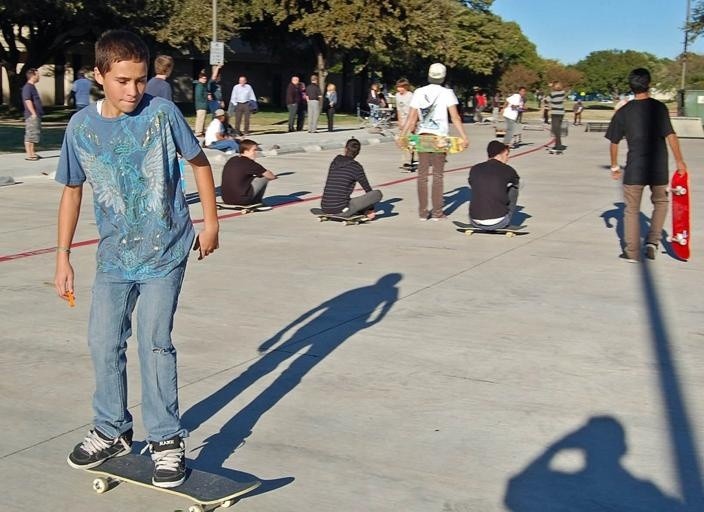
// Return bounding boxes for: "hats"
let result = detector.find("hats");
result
[427,63,446,84]
[215,109,224,116]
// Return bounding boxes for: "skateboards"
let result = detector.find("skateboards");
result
[216,202,263,214]
[83,453,263,512]
[399,160,419,171]
[665,170,690,262]
[452,220,528,237]
[551,144,567,155]
[503,143,518,149]
[309,208,366,226]
[394,134,465,153]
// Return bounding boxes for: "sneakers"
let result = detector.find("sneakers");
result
[619,254,638,263]
[140,437,187,487]
[645,244,656,259]
[67,427,133,470]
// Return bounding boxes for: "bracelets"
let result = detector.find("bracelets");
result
[57,247,72,255]
[610,165,619,172]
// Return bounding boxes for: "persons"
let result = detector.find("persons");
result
[144,54,176,100]
[399,62,470,222]
[221,139,277,211]
[473,80,585,150]
[66,69,92,113]
[53,28,221,489]
[193,65,416,158]
[467,139,520,231]
[320,139,382,220]
[603,68,686,263]
[22,68,45,163]
